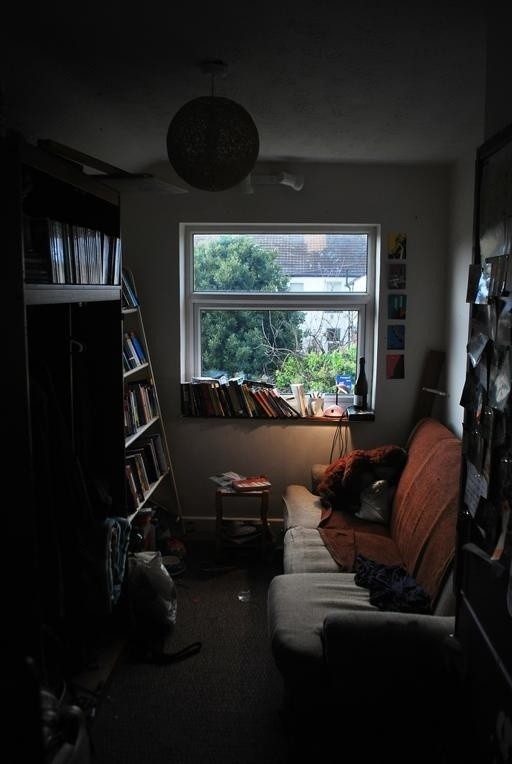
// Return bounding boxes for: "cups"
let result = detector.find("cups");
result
[308,398,325,417]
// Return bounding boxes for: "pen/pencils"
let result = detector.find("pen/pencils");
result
[310,392,321,399]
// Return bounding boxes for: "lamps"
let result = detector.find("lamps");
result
[165,57,260,192]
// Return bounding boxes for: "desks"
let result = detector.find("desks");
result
[215,483,271,553]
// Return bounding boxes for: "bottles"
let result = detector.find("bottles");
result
[352,358,368,410]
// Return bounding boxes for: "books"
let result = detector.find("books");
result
[182,373,303,419]
[120,273,147,374]
[124,434,168,508]
[234,476,273,491]
[124,378,159,438]
[208,469,247,486]
[25,219,122,286]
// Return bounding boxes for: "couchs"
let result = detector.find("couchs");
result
[266,415,464,725]
